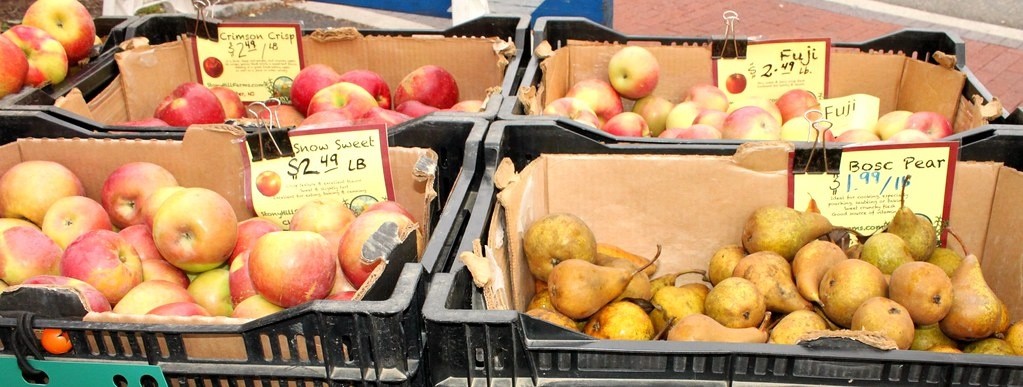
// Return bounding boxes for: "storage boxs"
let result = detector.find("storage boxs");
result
[0,12,1023,386]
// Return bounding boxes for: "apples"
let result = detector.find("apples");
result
[545,45,956,142]
[0,0,487,318]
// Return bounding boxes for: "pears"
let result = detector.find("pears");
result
[518,171,1022,359]
